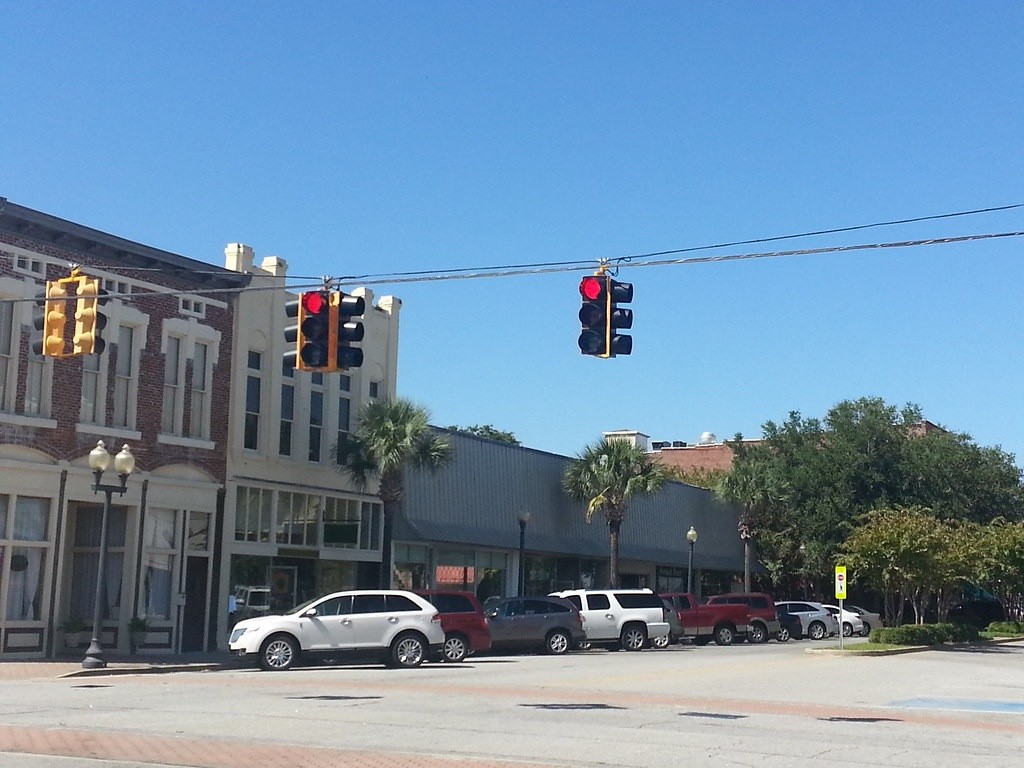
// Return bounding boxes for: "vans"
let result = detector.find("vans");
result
[703,593,782,645]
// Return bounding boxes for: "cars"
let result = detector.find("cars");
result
[661,598,685,646]
[773,601,836,641]
[821,603,864,637]
[843,605,884,636]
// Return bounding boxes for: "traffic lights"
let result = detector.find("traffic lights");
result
[300,289,333,369]
[332,291,366,370]
[607,275,634,359]
[282,292,302,371]
[32,281,67,357]
[577,276,608,358]
[75,277,110,357]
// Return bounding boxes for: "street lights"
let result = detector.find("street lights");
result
[79,437,137,669]
[515,505,530,612]
[686,524,697,593]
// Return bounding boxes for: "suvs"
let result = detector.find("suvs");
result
[546,588,670,652]
[408,588,493,664]
[226,588,447,669]
[479,594,587,657]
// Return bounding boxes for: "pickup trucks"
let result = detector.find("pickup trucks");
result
[656,592,749,646]
